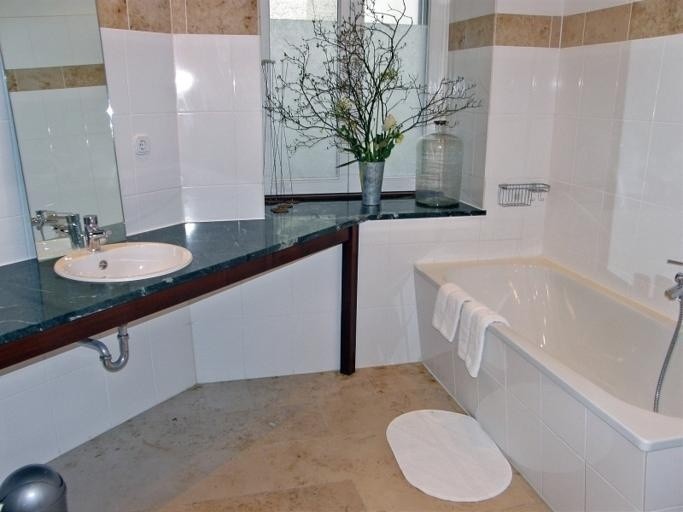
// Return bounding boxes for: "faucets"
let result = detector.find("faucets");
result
[31,209,85,250]
[77,215,105,252]
[663,271,683,302]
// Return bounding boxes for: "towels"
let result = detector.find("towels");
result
[455,298,508,378]
[428,282,470,344]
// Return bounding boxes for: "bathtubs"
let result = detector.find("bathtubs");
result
[413,256,683,511]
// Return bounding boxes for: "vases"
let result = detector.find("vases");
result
[355,157,384,208]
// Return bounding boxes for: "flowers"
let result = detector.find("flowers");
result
[260,1,480,162]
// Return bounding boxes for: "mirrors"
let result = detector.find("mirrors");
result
[1,0,126,264]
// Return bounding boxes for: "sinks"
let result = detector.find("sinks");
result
[32,235,75,264]
[50,240,192,284]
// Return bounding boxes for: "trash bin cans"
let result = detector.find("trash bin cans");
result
[0,463,68,512]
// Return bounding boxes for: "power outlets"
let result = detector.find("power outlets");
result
[133,135,150,155]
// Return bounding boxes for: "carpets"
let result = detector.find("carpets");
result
[380,405,514,503]
